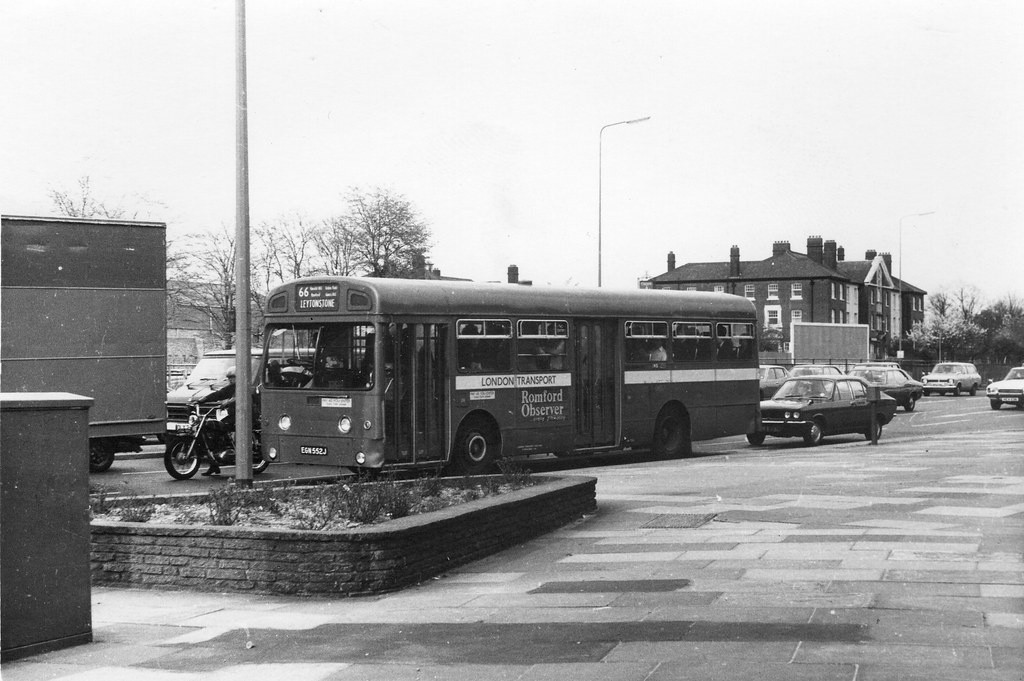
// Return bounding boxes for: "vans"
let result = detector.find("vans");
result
[157,348,366,446]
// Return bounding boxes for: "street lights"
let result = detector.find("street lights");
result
[899,210,936,351]
[598,113,651,290]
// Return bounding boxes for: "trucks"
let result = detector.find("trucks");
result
[1,215,167,471]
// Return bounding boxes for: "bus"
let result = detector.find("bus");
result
[256,276,760,478]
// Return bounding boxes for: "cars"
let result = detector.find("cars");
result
[851,362,901,382]
[845,368,923,412]
[757,364,789,400]
[985,363,1024,410]
[746,374,898,448]
[784,365,846,376]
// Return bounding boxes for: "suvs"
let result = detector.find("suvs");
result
[920,361,982,397]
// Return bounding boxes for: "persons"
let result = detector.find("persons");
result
[198,366,260,477]
[797,382,814,401]
[303,320,752,387]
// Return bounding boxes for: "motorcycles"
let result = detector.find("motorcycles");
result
[162,398,269,479]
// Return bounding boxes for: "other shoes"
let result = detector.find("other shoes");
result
[201,464,220,476]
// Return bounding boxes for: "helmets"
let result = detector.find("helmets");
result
[226,366,237,377]
